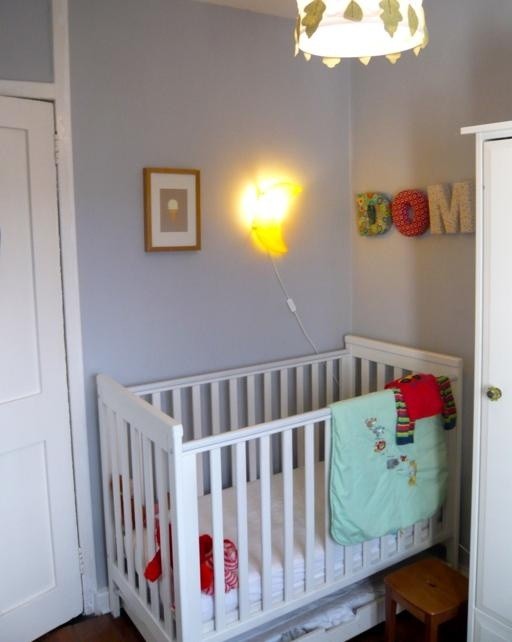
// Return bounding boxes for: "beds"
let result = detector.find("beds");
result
[96,333,463,641]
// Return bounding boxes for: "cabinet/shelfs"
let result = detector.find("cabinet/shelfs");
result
[459,121,511,641]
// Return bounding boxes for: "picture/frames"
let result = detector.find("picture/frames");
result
[143,167,201,251]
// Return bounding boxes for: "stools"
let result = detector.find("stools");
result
[382,556,469,642]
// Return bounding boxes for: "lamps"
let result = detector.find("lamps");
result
[294,1,429,69]
